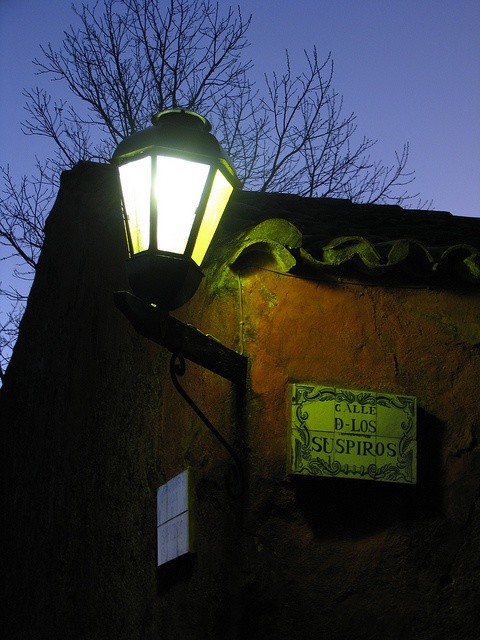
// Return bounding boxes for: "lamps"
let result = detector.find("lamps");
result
[91,109,248,384]
